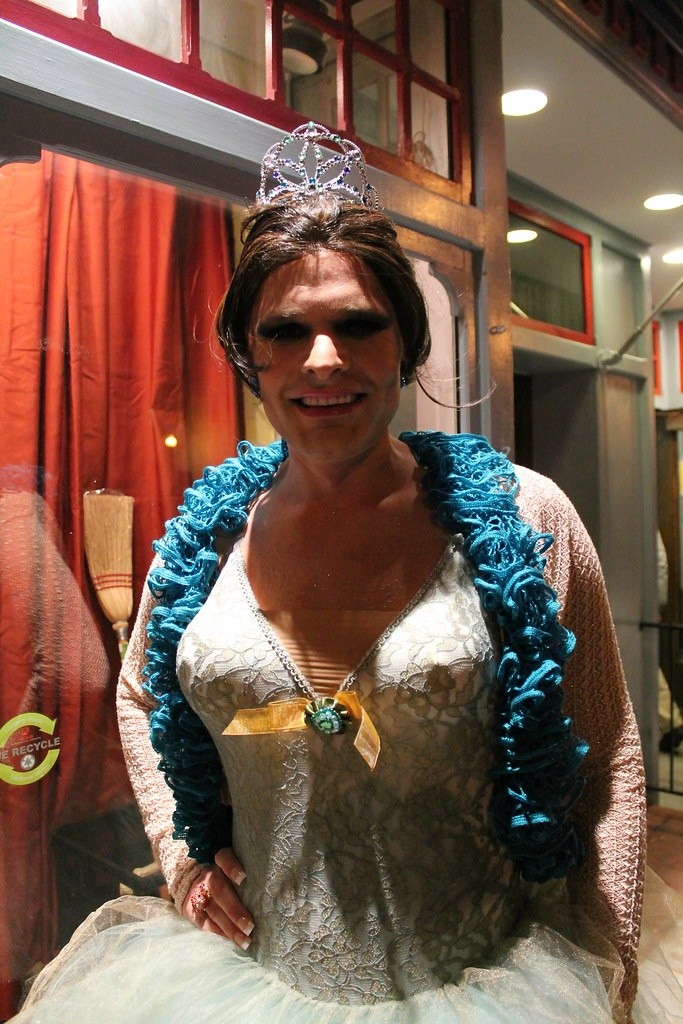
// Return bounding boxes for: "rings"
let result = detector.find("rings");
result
[191,883,213,912]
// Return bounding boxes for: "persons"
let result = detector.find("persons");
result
[0,119,647,1023]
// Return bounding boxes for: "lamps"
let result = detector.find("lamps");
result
[280,0,329,78]
